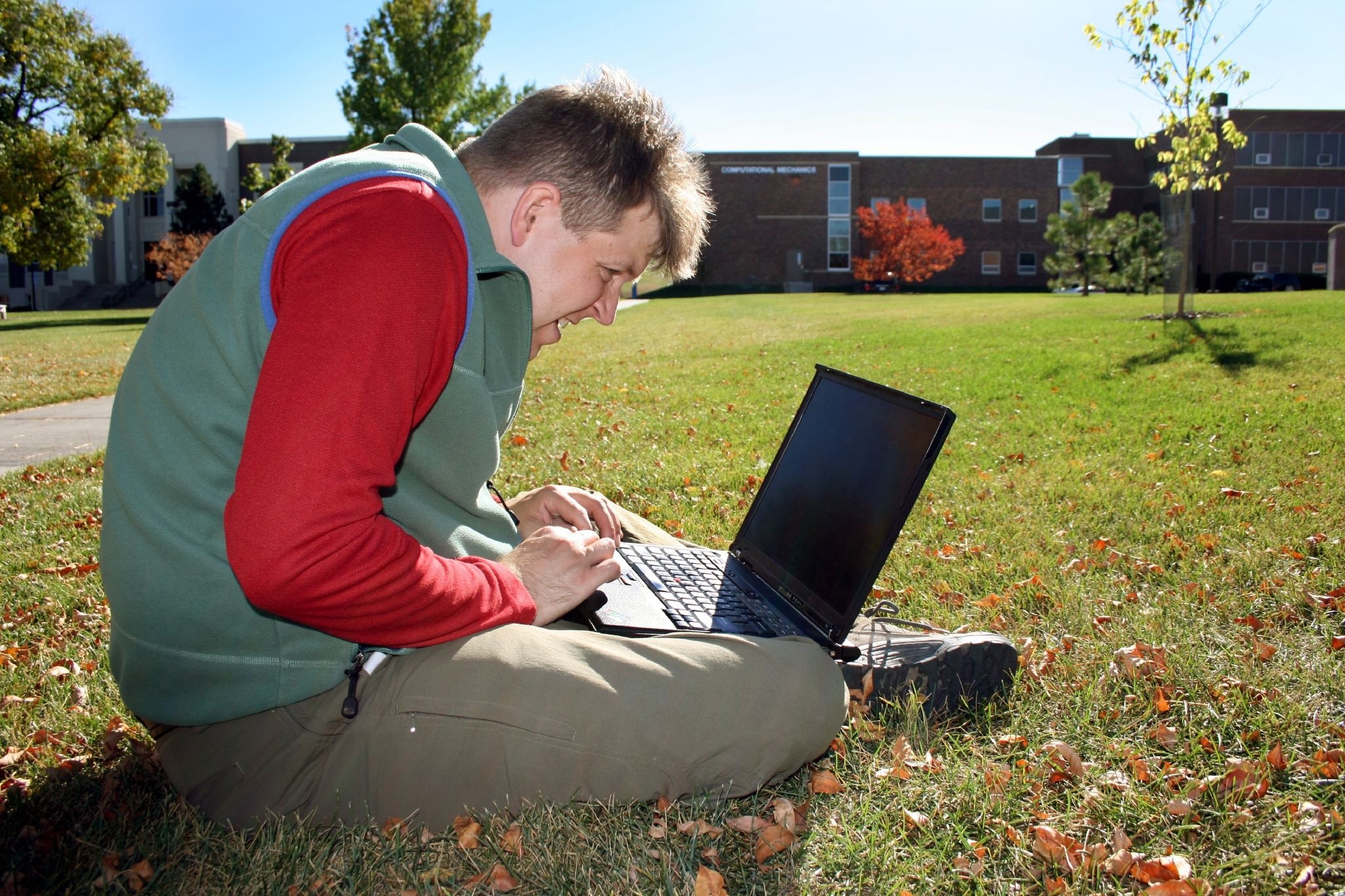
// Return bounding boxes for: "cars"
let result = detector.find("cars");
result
[864,271,899,293]
[1051,282,1105,293]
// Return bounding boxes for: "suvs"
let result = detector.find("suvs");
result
[1242,272,1300,292]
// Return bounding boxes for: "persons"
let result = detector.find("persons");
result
[98,62,1022,837]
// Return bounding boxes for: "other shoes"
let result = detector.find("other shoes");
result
[840,613,1019,725]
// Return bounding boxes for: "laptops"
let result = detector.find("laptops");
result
[570,363,957,665]
